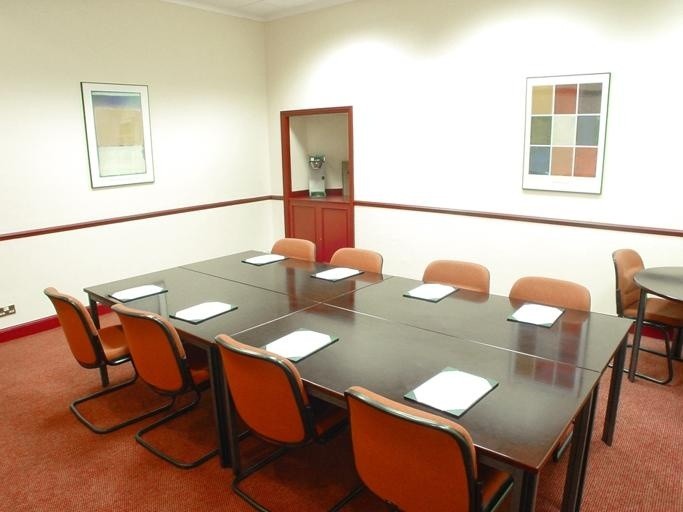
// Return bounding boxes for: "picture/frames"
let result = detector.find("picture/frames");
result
[80,79,158,190]
[521,70,613,197]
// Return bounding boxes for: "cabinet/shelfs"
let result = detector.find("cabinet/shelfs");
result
[286,192,353,262]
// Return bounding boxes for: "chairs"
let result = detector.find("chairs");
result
[269,235,319,265]
[607,242,681,387]
[214,333,367,512]
[40,280,175,433]
[423,260,490,294]
[509,276,591,462]
[324,243,385,276]
[343,386,516,512]
[110,303,254,469]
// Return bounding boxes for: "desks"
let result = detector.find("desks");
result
[627,265,682,386]
[214,303,601,512]
[178,250,396,303]
[83,267,322,469]
[322,276,637,512]
